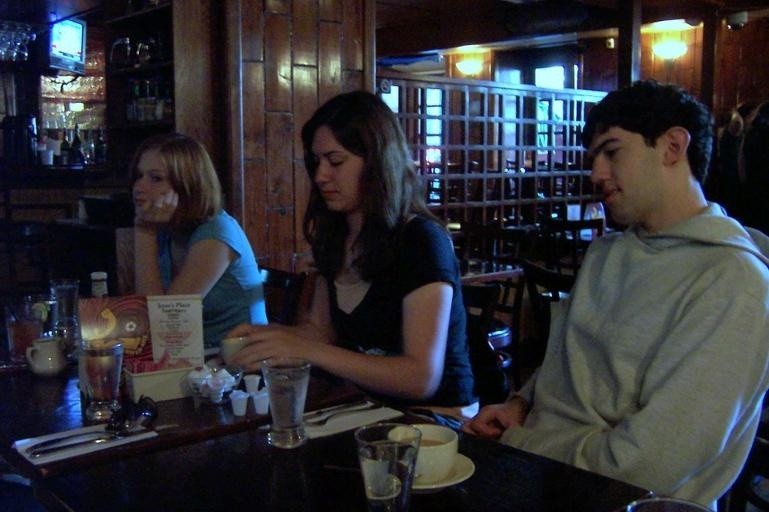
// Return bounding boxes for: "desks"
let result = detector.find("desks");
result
[1,324,374,486]
[38,397,709,511]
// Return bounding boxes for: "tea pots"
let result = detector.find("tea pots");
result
[26,337,67,377]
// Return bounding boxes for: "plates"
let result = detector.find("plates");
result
[387,453,476,492]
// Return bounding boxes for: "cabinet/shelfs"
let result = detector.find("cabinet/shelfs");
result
[41,22,177,167]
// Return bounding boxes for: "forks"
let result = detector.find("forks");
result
[304,403,382,427]
[29,410,158,459]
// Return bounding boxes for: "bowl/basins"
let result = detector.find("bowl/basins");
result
[387,424,461,485]
[183,366,244,406]
[221,337,249,363]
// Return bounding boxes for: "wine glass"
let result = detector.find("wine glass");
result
[0,19,37,63]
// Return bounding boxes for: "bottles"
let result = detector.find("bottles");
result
[122,75,177,124]
[24,113,112,175]
[89,272,109,298]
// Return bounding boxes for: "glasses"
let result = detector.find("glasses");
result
[107,395,157,429]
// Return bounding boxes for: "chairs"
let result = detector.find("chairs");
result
[717,431,768,512]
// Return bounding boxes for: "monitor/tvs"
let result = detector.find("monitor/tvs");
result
[45,10,88,78]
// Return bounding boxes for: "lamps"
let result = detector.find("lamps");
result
[651,38,689,84]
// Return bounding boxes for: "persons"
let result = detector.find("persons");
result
[457,78,769,512]
[224,90,479,432]
[128,133,269,368]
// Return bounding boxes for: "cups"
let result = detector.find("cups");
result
[24,293,60,339]
[229,391,249,417]
[2,302,43,366]
[258,356,313,451]
[49,278,81,333]
[253,390,270,416]
[75,338,125,423]
[353,420,422,512]
[243,373,262,393]
